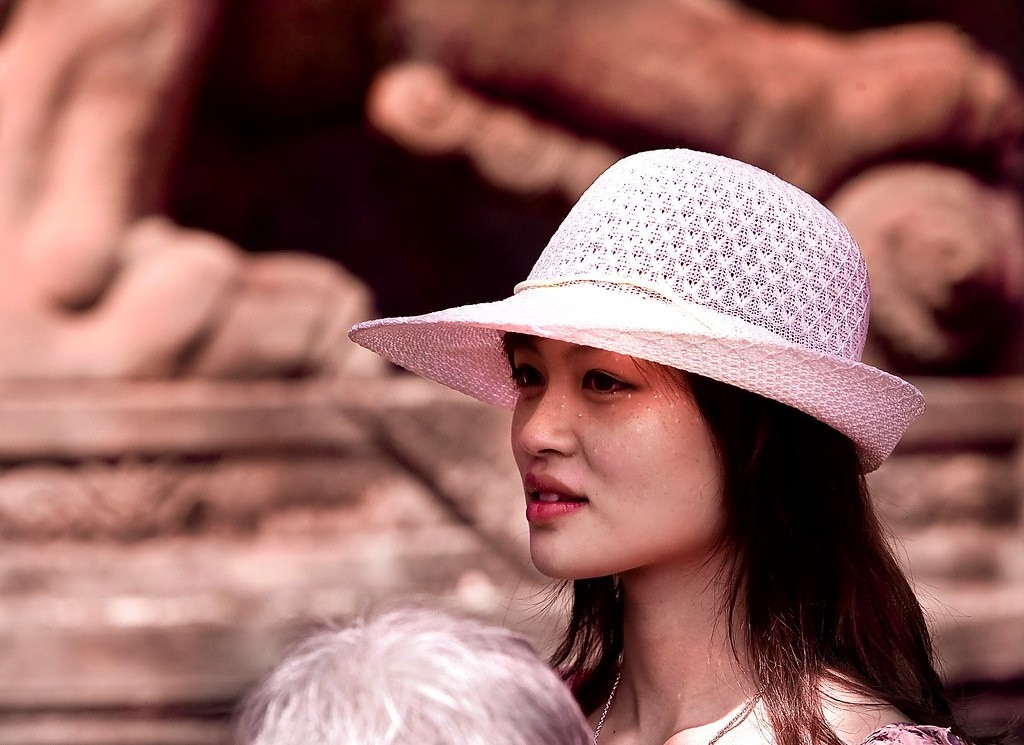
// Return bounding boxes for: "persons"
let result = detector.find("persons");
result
[231,605,593,745]
[348,149,966,745]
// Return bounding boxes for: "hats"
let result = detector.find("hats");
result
[346,147,929,473]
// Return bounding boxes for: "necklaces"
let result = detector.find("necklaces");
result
[594,651,766,745]
